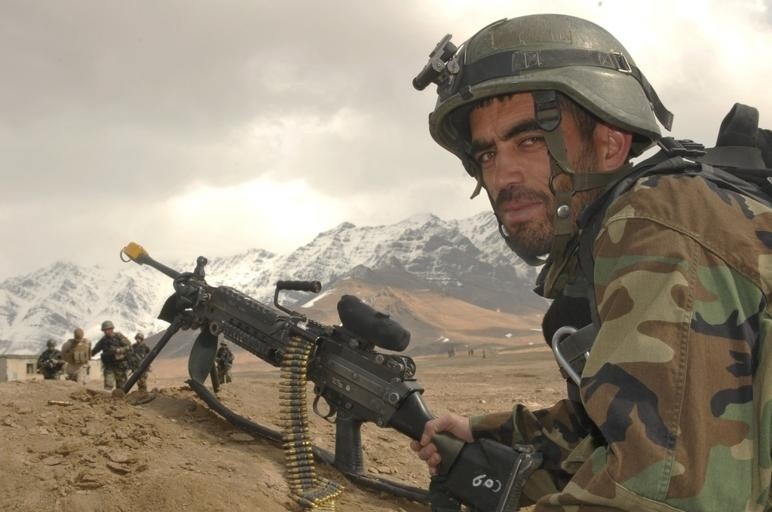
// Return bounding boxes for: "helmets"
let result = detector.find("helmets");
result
[220,340,227,345]
[47,339,57,346]
[102,322,114,330]
[74,329,83,340]
[136,333,144,341]
[428,15,662,187]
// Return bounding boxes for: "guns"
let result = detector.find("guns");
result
[117,242,542,512]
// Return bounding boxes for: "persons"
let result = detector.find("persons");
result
[411,15,772,512]
[215,342,233,384]
[35,321,151,395]
